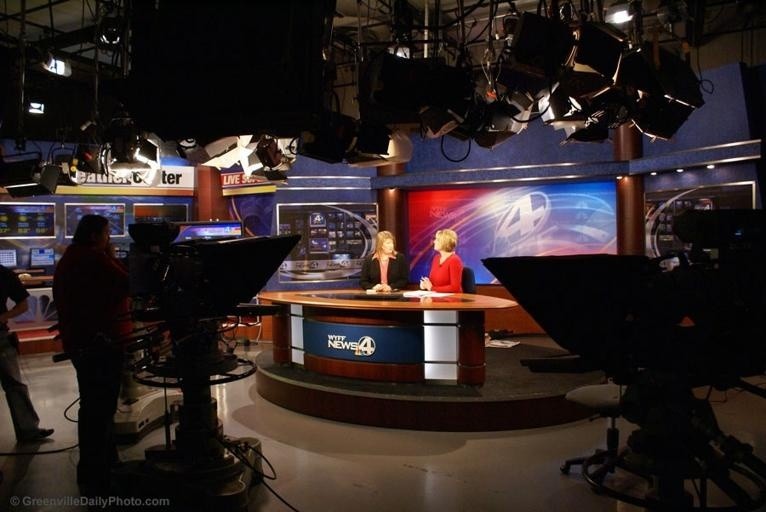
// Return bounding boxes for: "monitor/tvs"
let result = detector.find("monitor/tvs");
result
[170,220,244,243]
[133,203,189,224]
[0,201,56,240]
[64,203,126,238]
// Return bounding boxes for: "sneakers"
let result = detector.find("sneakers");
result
[20,429,54,439]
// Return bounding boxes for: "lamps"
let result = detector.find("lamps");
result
[0,0,714,198]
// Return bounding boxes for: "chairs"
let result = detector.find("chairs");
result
[462,268,476,294]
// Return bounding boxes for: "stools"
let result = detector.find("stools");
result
[559,384,654,495]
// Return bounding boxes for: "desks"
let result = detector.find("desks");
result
[257,288,518,386]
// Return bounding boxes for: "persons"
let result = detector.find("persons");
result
[106,238,139,401]
[419,229,463,291]
[0,262,53,444]
[51,213,134,497]
[359,231,410,288]
